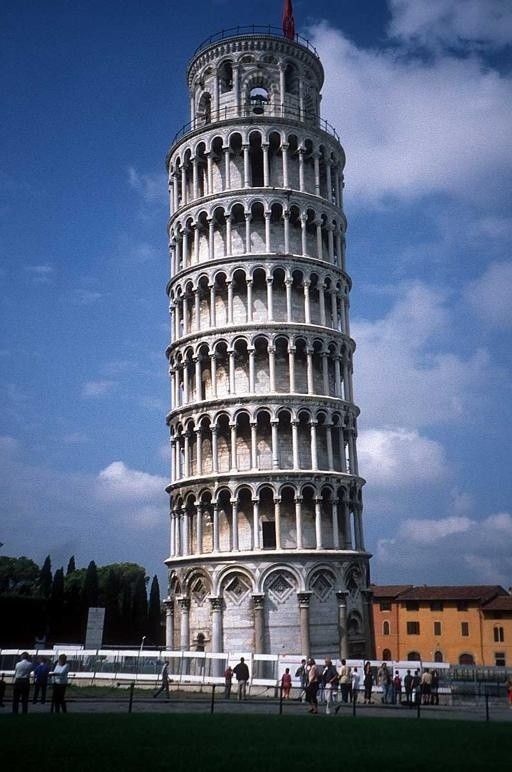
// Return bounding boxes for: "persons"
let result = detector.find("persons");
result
[223,657,441,714]
[506,677,511,708]
[152,660,173,699]
[0,650,71,714]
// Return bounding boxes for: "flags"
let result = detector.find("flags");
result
[282,0,295,41]
